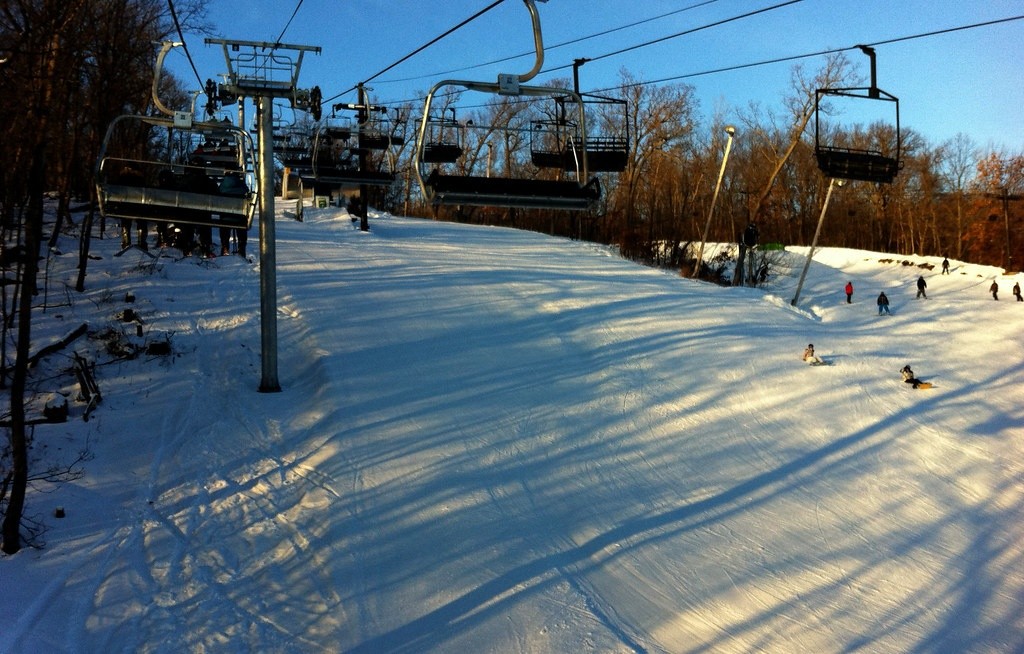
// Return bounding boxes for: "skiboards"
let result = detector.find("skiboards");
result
[916,382,932,389]
[811,360,834,366]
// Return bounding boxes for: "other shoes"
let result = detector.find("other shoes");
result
[916,297,920,300]
[924,297,927,299]
[185,246,217,259]
[221,245,230,256]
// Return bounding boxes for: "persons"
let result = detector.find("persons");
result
[901,364,926,389]
[844,281,853,303]
[916,275,927,299]
[988,279,999,300]
[876,291,890,316]
[941,255,950,274]
[802,343,817,366]
[1012,281,1023,301]
